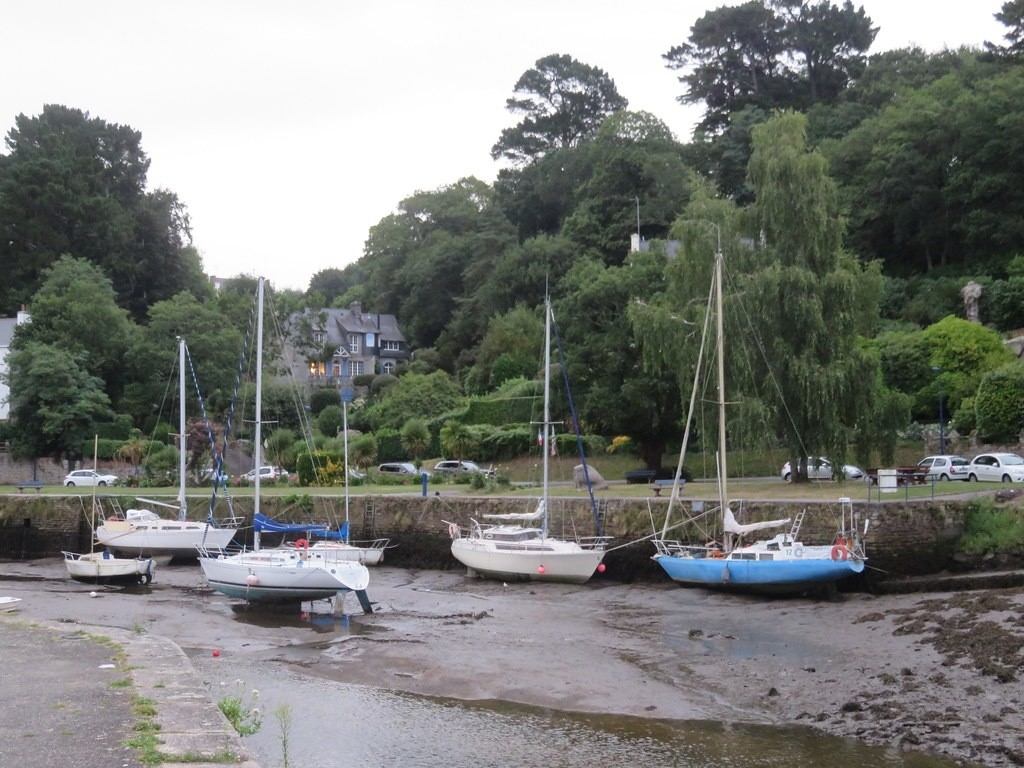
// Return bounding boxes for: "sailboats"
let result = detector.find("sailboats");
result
[649,244,871,586]
[96,337,244,555]
[194,273,392,604]
[442,280,614,584]
[53,435,158,582]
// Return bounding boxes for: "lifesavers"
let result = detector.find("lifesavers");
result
[830,544,848,561]
[295,537,308,550]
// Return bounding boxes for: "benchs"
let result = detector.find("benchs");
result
[16,480,43,494]
[625,470,656,484]
[864,464,930,485]
[650,480,685,496]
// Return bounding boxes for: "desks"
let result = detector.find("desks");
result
[898,468,917,485]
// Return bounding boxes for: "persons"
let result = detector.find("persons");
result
[962,281,981,323]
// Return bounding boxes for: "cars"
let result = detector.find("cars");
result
[377,462,433,481]
[435,461,494,479]
[240,465,290,482]
[968,452,1024,483]
[64,469,120,486]
[915,454,972,483]
[196,469,229,485]
[780,454,864,482]
